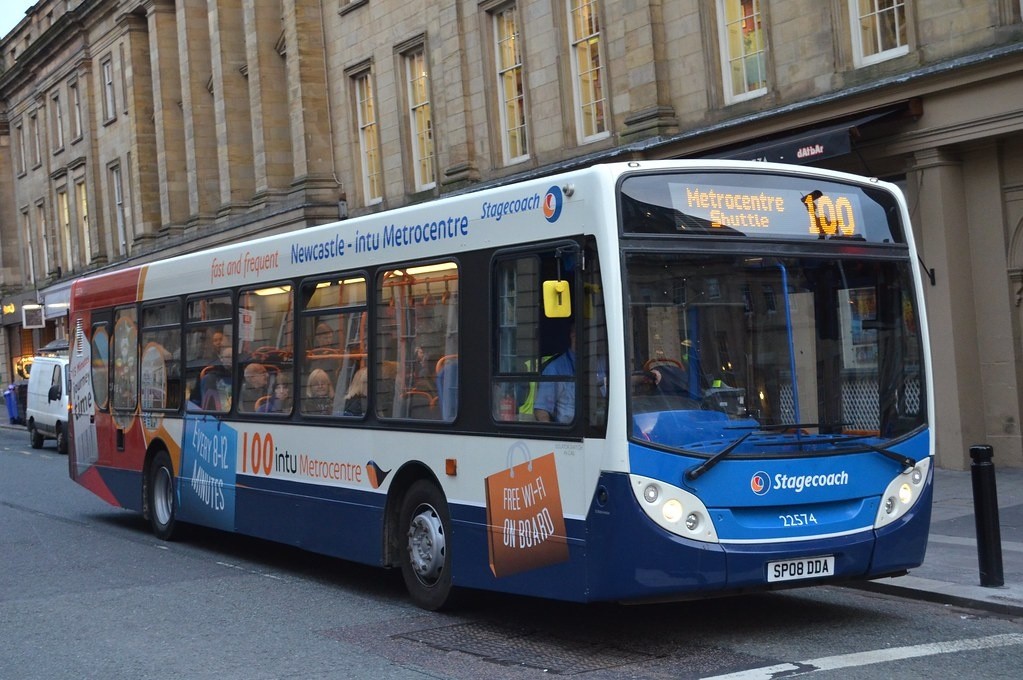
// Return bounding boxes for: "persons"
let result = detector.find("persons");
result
[518,318,662,426]
[190,323,441,419]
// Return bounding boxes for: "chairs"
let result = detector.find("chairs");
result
[645,358,688,396]
[511,345,570,419]
[199,346,458,422]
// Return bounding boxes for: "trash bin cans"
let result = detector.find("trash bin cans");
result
[14,381,28,426]
[3,384,19,425]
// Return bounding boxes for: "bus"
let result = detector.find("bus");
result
[66,155,936,613]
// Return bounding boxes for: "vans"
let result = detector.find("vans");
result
[26,355,71,454]
